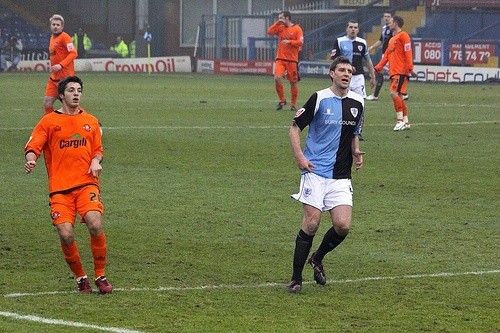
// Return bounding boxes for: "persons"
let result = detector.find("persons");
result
[332,20,377,140]
[266,11,304,112]
[366,12,409,102]
[71,28,92,59]
[24,76,112,294]
[43,14,78,115]
[287,57,366,294]
[369,15,417,131]
[110,36,129,58]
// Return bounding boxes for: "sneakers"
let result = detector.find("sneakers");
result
[400,123,410,130]
[291,107,296,111]
[308,253,326,285]
[401,95,409,100]
[94,274,112,294]
[366,94,378,100]
[74,274,92,294]
[288,280,302,293]
[394,122,405,130]
[276,100,287,110]
